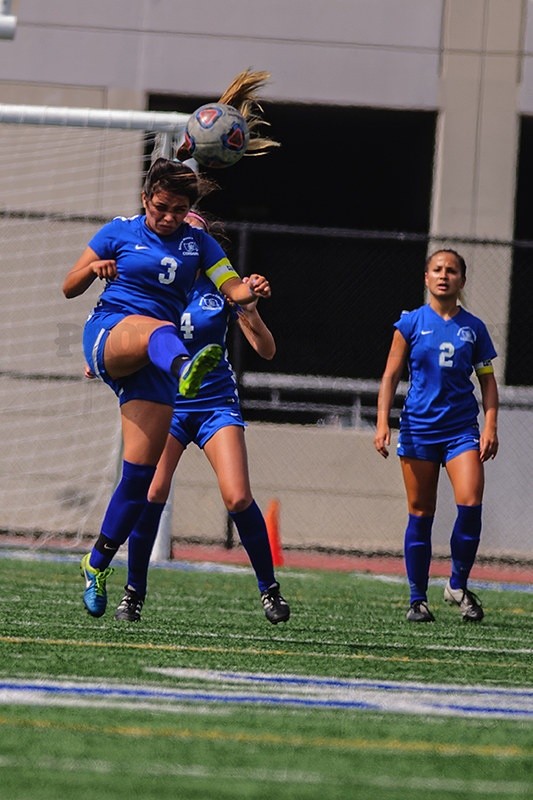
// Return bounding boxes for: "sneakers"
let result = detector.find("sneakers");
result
[115,585,145,623]
[261,582,290,624]
[405,600,436,622]
[444,578,484,622]
[178,344,223,398]
[80,552,114,618]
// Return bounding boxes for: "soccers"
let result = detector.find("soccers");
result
[184,104,249,167]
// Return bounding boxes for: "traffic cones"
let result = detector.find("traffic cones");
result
[265,497,285,567]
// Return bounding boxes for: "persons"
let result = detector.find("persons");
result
[112,208,294,627]
[62,68,284,620]
[374,250,501,620]
